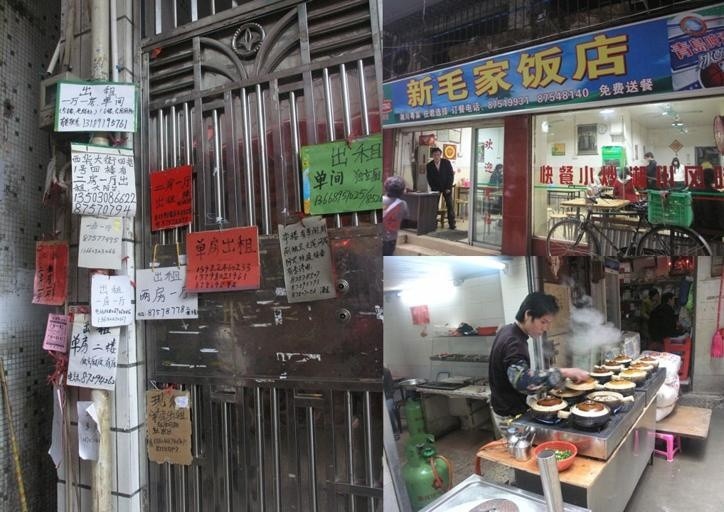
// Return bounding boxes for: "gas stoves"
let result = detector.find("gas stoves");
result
[508,366,669,461]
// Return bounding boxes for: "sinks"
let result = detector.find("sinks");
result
[417,380,462,390]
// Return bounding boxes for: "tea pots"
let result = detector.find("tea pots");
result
[506,426,538,461]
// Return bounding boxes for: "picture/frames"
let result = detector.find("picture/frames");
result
[576,124,598,156]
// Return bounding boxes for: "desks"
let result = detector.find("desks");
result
[397,190,440,235]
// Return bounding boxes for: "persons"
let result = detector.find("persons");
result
[426,145,457,229]
[613,166,637,203]
[592,170,610,186]
[639,288,661,338]
[490,164,503,215]
[649,292,675,341]
[699,153,715,180]
[382,176,409,255]
[669,157,686,181]
[487,291,590,438]
[643,151,657,184]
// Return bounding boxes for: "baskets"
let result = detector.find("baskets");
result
[646,190,692,228]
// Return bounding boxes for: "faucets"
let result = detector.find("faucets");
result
[434,369,451,384]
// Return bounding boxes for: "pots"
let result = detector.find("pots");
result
[527,355,661,432]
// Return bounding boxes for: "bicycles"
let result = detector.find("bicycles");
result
[543,187,713,256]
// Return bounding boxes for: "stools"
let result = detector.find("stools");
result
[663,335,691,380]
[434,208,446,230]
[653,430,682,461]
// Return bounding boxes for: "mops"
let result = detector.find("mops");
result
[711,265,724,358]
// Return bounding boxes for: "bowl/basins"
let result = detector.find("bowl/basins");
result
[476,326,498,336]
[399,378,428,391]
[536,441,578,473]
